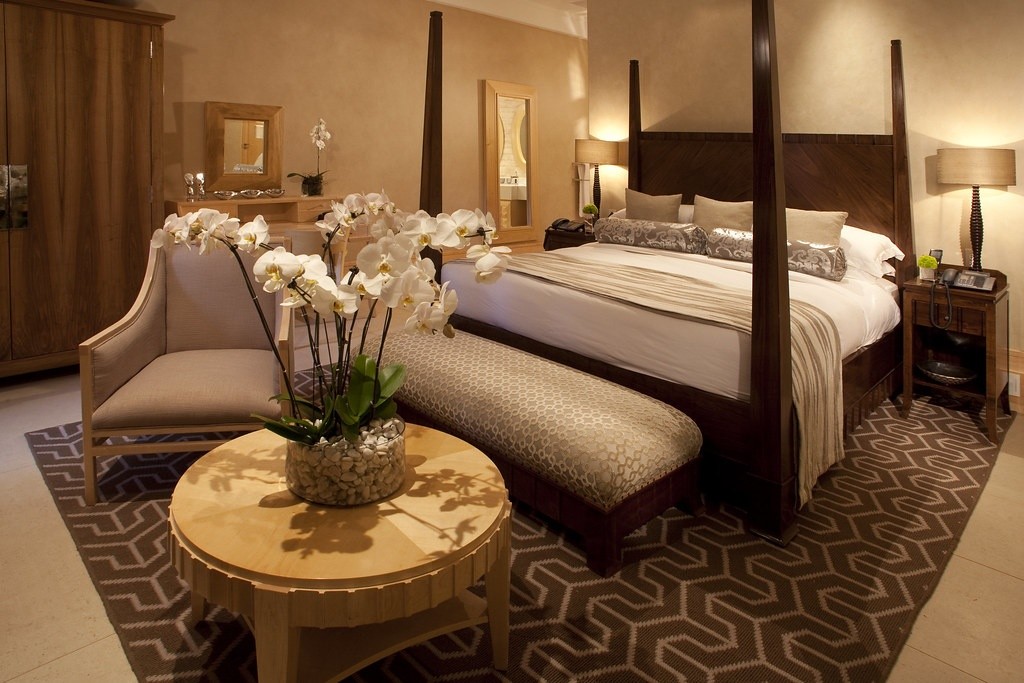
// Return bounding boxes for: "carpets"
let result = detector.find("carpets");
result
[23,361,1017,683]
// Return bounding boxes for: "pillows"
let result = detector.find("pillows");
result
[594,188,905,282]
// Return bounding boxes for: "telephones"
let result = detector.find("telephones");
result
[553,218,584,232]
[939,268,997,291]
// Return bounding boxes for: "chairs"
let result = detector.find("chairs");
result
[78,237,295,505]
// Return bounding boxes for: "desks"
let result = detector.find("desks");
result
[168,423,512,683]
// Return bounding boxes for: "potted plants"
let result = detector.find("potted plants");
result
[583,202,598,219]
[917,255,937,280]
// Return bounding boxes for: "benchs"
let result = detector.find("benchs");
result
[349,329,704,577]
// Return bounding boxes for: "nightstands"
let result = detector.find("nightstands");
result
[544,230,595,251]
[900,264,1011,442]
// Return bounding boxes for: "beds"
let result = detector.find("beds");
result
[419,0,915,547]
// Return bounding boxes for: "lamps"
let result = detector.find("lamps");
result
[936,148,1016,273]
[575,139,619,219]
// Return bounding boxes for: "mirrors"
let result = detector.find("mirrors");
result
[482,79,540,244]
[204,101,285,191]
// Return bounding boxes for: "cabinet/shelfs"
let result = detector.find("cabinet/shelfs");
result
[0,0,175,378]
[166,195,345,232]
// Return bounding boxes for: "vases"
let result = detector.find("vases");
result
[302,184,323,196]
[286,412,407,507]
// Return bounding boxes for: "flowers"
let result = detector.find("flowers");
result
[150,188,512,443]
[287,118,331,184]
